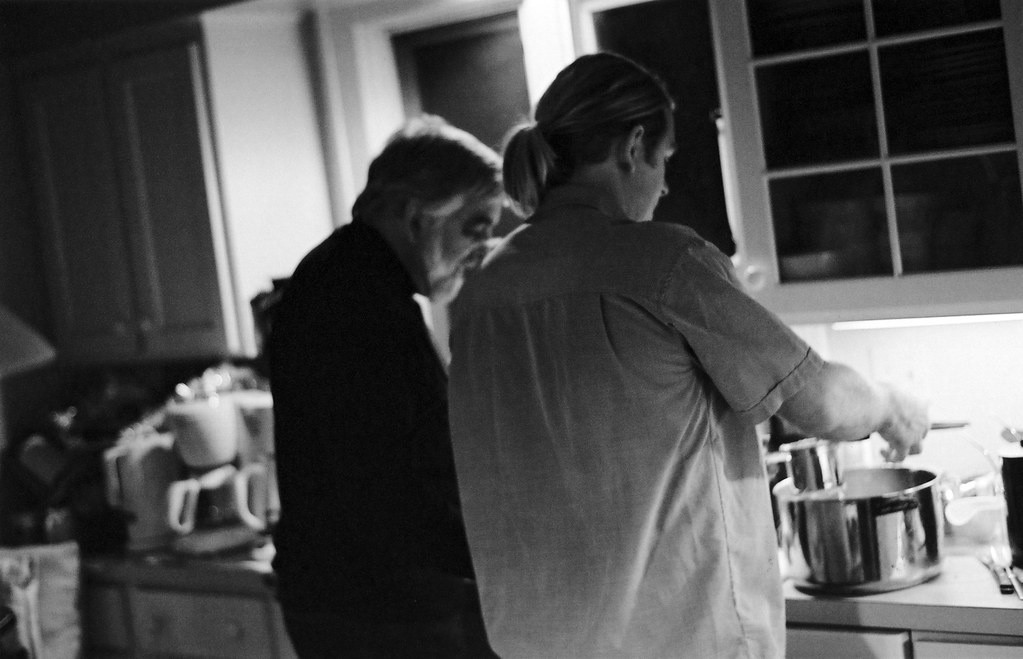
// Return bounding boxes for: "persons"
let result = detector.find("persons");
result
[443,54,931,659]
[249,118,503,659]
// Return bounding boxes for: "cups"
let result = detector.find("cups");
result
[165,391,282,549]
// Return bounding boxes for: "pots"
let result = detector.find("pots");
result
[773,467,948,595]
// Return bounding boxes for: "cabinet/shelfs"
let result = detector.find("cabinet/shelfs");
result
[17,0,327,371]
[80,590,295,658]
[787,624,1023,659]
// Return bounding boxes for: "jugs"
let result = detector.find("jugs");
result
[103,429,179,553]
